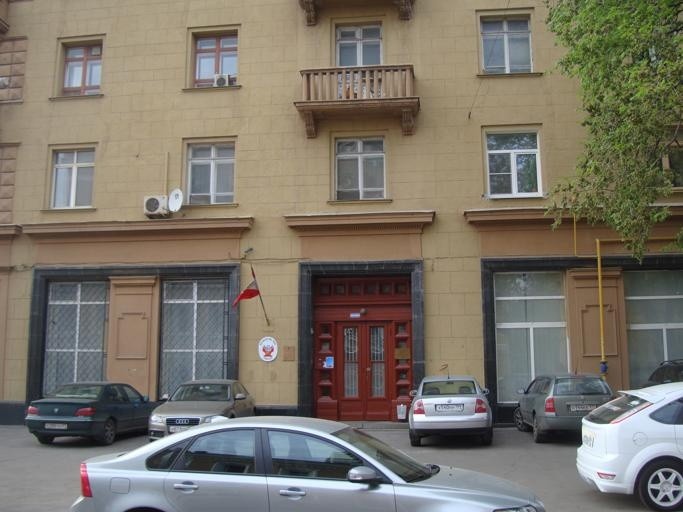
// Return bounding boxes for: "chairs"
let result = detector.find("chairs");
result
[424,386,471,395]
[79,388,120,401]
[193,441,320,476]
[189,386,226,401]
[559,382,590,394]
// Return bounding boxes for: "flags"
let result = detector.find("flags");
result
[230,280,259,307]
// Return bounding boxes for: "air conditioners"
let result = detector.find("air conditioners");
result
[142,194,169,220]
[213,74,229,88]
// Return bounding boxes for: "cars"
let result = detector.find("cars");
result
[149,378,255,444]
[26,382,157,445]
[512,373,612,445]
[577,357,678,510]
[409,372,493,447]
[71,415,545,512]
